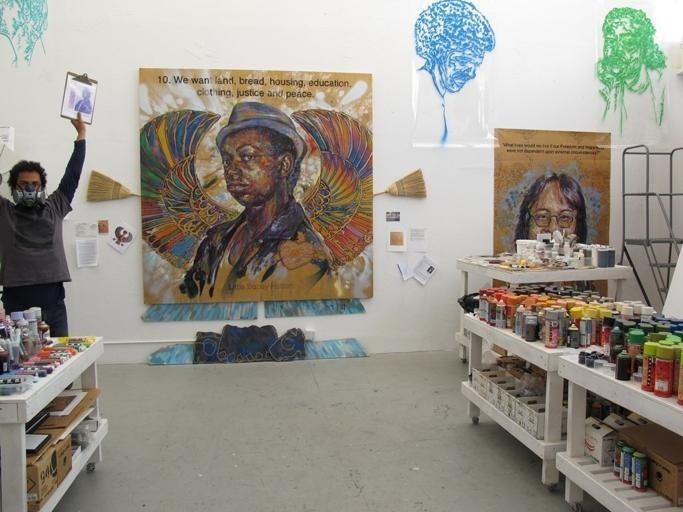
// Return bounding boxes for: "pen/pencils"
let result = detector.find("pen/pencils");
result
[0,336,94,385]
[509,264,530,268]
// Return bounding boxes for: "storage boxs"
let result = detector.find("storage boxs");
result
[616,424,682,507]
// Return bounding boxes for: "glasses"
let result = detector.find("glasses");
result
[527,209,574,228]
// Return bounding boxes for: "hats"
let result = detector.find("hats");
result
[215,102,307,163]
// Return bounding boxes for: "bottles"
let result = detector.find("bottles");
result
[611,439,649,492]
[477,283,682,406]
[0,346,9,374]
[11,306,50,342]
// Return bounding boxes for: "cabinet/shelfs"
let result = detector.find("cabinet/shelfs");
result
[1,336,109,512]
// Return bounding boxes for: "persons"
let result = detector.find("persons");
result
[515,171,587,252]
[0,113,86,339]
[74,87,91,113]
[176,101,344,300]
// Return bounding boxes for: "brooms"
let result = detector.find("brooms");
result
[86,170,141,204]
[372,170,427,201]
